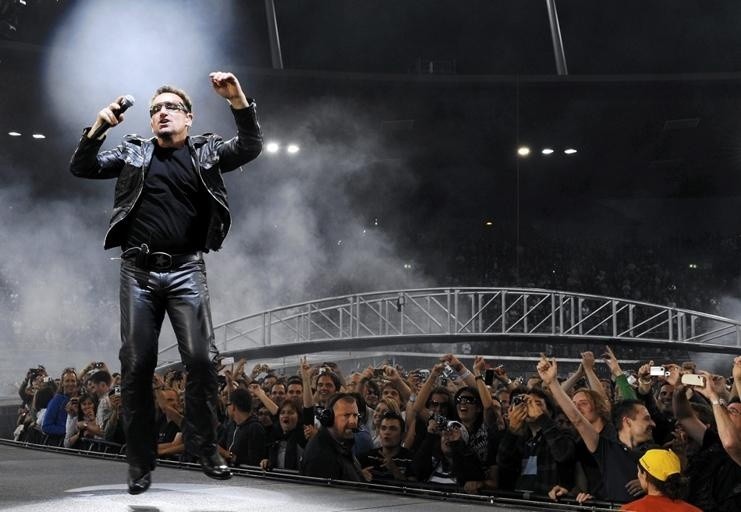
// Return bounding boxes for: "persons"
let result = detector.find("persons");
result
[16,218,741,512]
[68,71,266,496]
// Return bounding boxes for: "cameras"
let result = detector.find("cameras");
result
[30,368,39,379]
[95,361,103,367]
[442,364,453,378]
[373,368,384,376]
[418,369,430,377]
[43,376,52,383]
[434,415,447,431]
[114,386,121,396]
[318,366,327,375]
[511,395,525,404]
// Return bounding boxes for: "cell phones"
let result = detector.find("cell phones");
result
[220,356,235,365]
[681,373,706,388]
[485,368,493,387]
[650,366,665,377]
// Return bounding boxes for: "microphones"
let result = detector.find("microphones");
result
[91,94,136,139]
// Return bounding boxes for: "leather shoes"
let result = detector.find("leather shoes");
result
[183,442,233,479]
[128,458,156,494]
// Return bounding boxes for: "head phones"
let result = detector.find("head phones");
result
[320,393,362,428]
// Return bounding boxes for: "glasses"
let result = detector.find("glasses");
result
[455,395,477,404]
[431,400,449,410]
[151,102,188,118]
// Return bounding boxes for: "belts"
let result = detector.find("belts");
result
[121,247,202,268]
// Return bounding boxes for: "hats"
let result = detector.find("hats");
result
[631,447,681,482]
[448,421,469,444]
